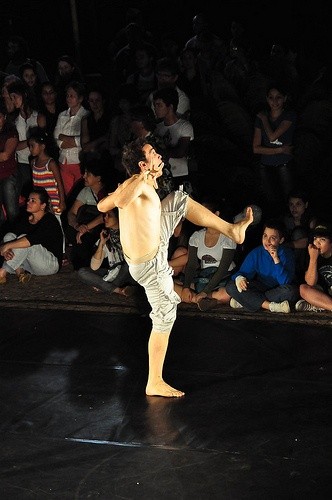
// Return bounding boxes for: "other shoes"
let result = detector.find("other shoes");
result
[198,297,218,312]
[123,286,137,296]
[0,277,9,285]
[18,273,32,284]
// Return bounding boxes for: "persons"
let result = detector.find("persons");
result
[0,0,332,314]
[97,139,254,398]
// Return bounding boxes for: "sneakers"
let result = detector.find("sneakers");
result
[295,300,325,311]
[269,300,290,314]
[230,297,243,309]
